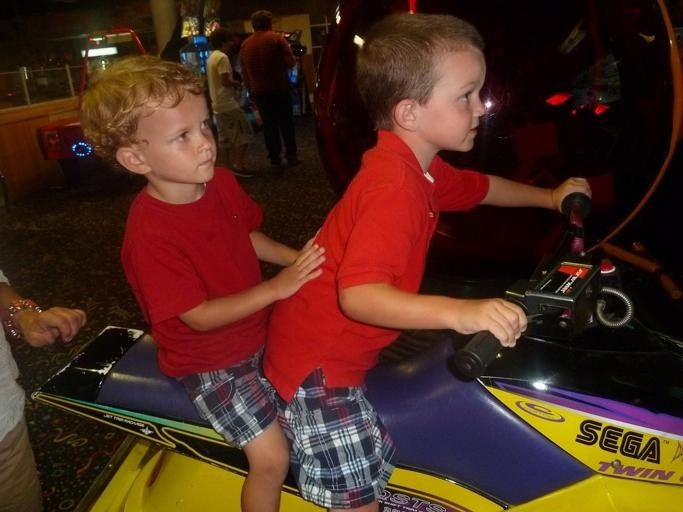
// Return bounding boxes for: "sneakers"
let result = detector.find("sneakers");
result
[231,155,304,178]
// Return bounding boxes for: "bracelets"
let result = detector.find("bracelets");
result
[1,298,41,341]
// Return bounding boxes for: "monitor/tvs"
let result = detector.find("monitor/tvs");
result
[288,66,298,83]
[181,50,209,74]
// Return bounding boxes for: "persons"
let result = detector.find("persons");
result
[237,11,306,166]
[208,30,255,178]
[258,12,590,510]
[0,271,85,511]
[81,55,325,511]
